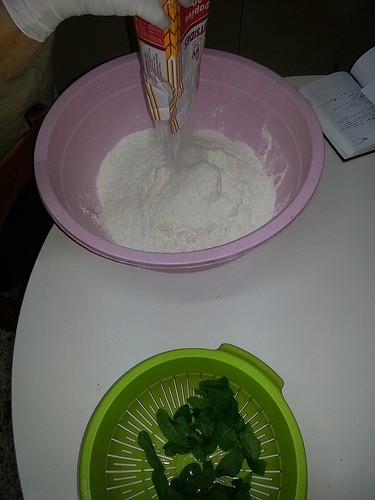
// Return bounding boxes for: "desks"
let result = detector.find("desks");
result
[11,73,375,500]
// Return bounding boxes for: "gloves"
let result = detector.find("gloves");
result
[2,0,193,43]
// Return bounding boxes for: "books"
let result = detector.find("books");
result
[298,48,375,162]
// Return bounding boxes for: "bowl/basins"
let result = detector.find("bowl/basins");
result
[34,50,325,273]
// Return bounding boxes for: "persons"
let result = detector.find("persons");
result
[0,0,192,160]
